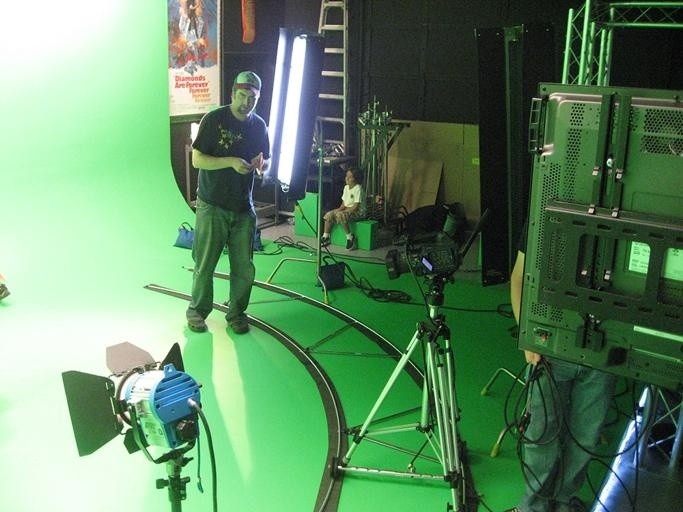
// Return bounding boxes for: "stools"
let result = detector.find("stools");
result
[329,219,378,251]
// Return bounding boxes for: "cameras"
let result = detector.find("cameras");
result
[384,242,459,280]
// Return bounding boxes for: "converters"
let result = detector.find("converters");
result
[368,292,383,298]
[384,294,400,299]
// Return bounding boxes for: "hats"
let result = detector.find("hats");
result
[233,71,262,96]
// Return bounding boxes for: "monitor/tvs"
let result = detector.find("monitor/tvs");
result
[516,80,683,394]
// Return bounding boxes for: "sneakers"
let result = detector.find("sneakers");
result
[345,232,356,250]
[319,235,330,247]
[186,319,207,333]
[228,316,250,336]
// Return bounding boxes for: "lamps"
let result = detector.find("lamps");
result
[261,29,323,201]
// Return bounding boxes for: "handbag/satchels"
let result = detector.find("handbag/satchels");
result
[315,254,346,292]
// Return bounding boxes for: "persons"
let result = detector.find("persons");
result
[318,166,366,251]
[504,210,617,512]
[183,69,269,333]
